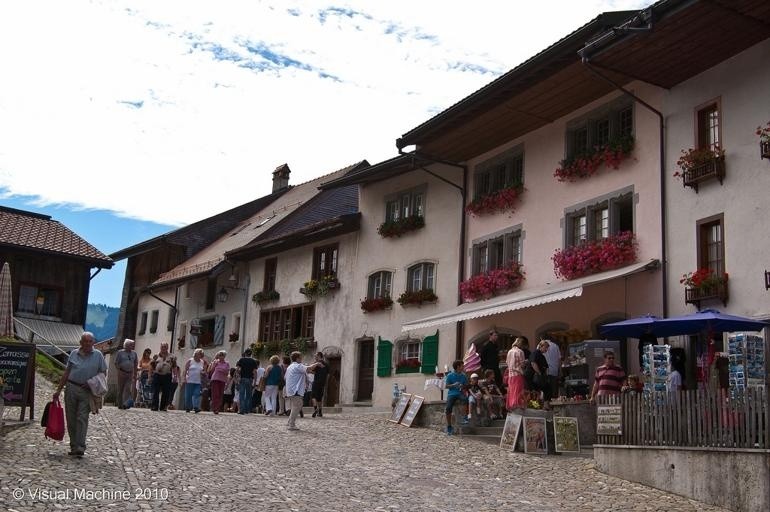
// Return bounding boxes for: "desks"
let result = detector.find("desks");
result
[424,378,447,400]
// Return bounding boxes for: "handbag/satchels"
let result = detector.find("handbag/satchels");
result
[41,396,64,441]
[256,377,265,392]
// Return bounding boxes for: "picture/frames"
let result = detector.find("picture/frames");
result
[523,416,548,454]
[596,403,623,436]
[389,392,412,423]
[499,412,522,450]
[400,394,425,427]
[553,416,580,453]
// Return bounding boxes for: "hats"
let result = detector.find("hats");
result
[470,372,479,378]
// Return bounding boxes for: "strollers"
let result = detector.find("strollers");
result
[134,368,156,409]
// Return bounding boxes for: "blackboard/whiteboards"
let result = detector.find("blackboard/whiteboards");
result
[388,393,425,428]
[0,341,36,406]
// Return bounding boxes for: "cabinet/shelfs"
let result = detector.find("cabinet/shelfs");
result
[558,341,621,397]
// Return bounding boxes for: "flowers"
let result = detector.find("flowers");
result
[458,258,523,303]
[549,229,640,279]
[755,118,770,143]
[359,286,394,315]
[555,129,637,181]
[672,142,725,182]
[465,177,529,223]
[679,262,732,304]
[303,269,343,300]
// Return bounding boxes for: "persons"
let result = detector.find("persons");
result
[443,330,563,436]
[589,351,632,406]
[668,359,683,391]
[623,374,639,396]
[53,331,108,455]
[113,338,328,432]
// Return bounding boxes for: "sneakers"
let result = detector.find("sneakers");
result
[151,407,158,411]
[476,407,481,415]
[464,414,472,424]
[160,407,167,411]
[194,407,201,413]
[285,422,300,430]
[186,408,190,412]
[214,409,291,416]
[68,451,84,455]
[489,413,504,420]
[299,409,303,418]
[312,409,322,417]
[447,426,454,435]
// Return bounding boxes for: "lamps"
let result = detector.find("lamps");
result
[215,284,245,303]
[227,267,239,282]
